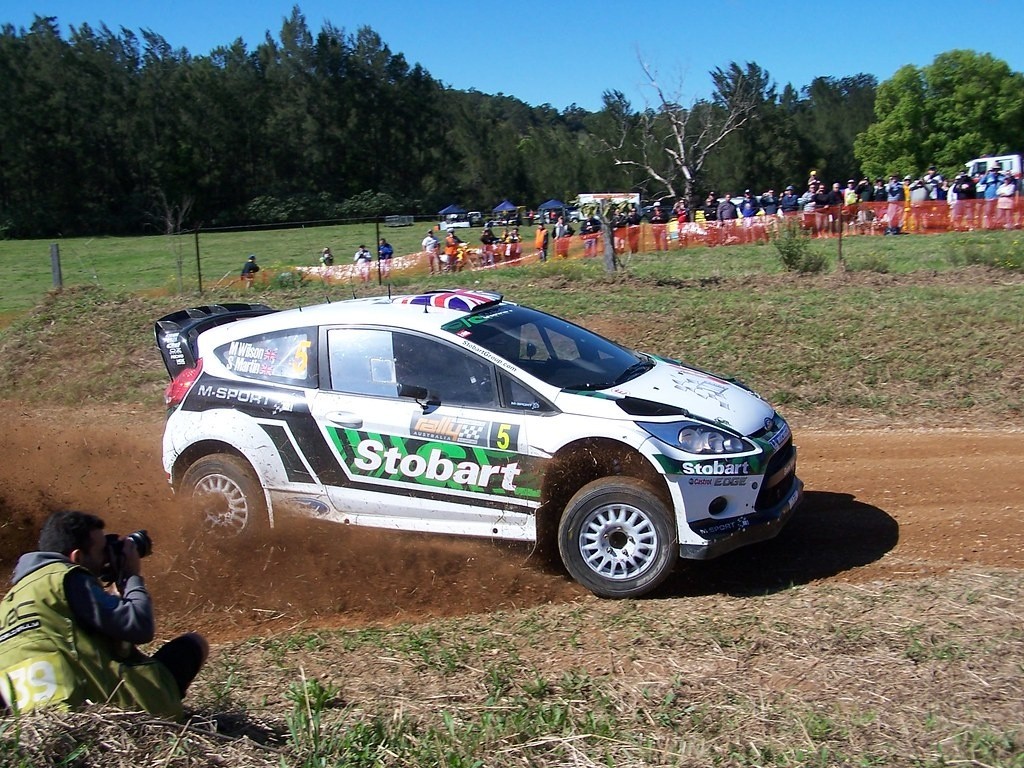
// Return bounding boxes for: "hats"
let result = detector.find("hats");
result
[428,229,433,231]
[539,222,543,225]
[654,202,660,206]
[904,175,911,180]
[864,177,869,181]
[785,187,788,191]
[787,186,794,191]
[447,228,455,232]
[928,166,936,170]
[745,189,751,193]
[810,171,816,175]
[876,177,884,181]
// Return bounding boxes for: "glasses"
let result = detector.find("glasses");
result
[710,194,715,196]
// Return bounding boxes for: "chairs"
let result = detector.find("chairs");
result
[390,334,440,402]
[434,339,489,404]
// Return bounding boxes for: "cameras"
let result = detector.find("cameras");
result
[101,529,153,581]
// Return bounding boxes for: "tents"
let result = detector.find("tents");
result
[439,205,468,215]
[538,199,578,211]
[494,201,517,212]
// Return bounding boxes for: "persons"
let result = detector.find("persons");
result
[648,201,670,251]
[422,230,442,274]
[580,213,602,257]
[0,512,209,721]
[515,212,521,226]
[354,245,372,282]
[321,247,334,268]
[529,209,534,226]
[379,238,393,277]
[612,208,641,254]
[241,255,260,280]
[671,199,690,248]
[535,223,549,262]
[704,162,1024,248]
[480,227,522,266]
[552,215,575,260]
[445,228,463,272]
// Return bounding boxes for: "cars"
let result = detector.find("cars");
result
[569,206,673,224]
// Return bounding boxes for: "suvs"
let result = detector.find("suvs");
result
[467,212,482,227]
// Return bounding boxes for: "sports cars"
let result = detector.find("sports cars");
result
[155,289,805,600]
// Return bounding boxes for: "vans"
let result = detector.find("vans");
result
[964,154,1022,192]
